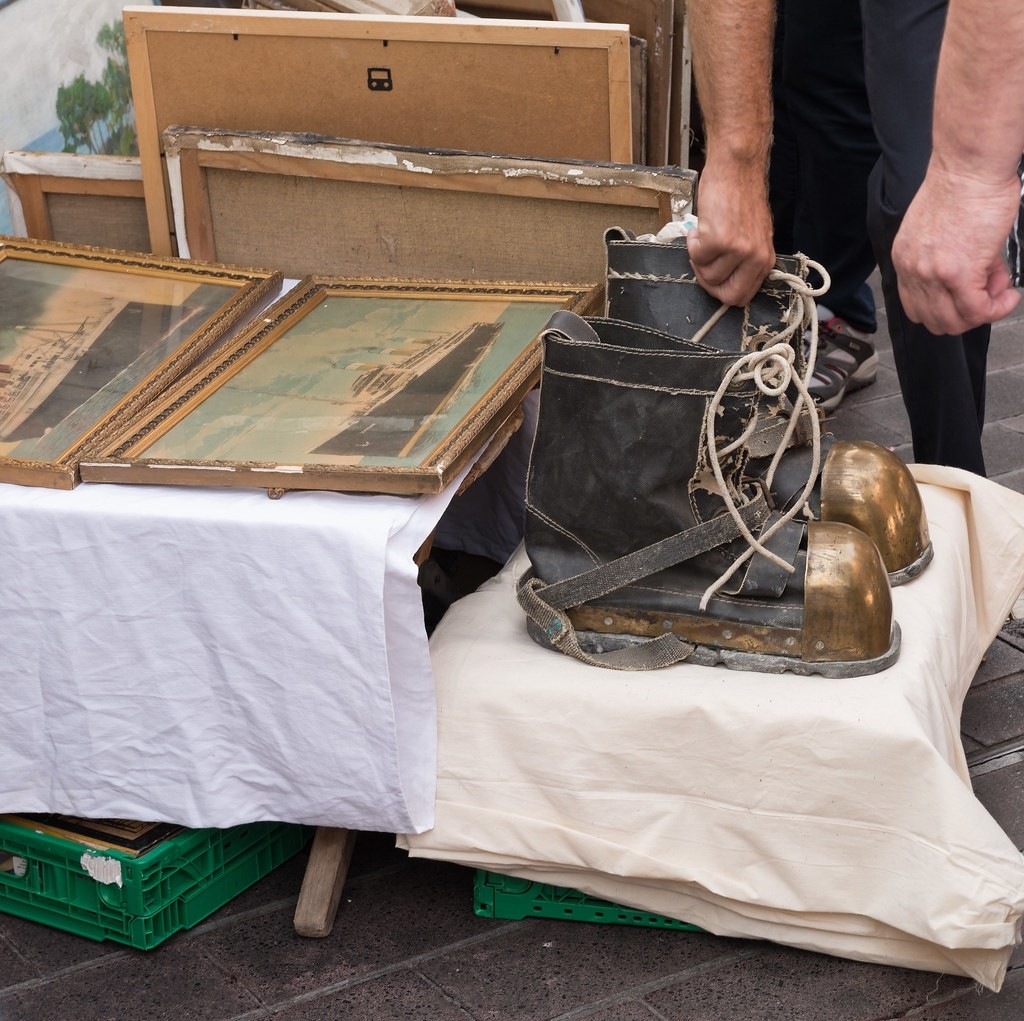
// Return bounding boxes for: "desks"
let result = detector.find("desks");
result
[0,404,529,942]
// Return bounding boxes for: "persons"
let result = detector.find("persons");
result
[680,0,1024,476]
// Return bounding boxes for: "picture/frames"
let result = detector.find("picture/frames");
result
[78,273,608,494]
[0,232,285,492]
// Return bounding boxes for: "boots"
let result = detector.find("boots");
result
[602,224,936,588]
[513,310,902,676]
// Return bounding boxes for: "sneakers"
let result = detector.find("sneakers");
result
[805,317,880,413]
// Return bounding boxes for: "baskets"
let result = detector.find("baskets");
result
[0,811,308,953]
[474,864,707,932]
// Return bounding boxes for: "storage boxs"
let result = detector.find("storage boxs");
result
[469,864,709,932]
[0,814,317,952]
